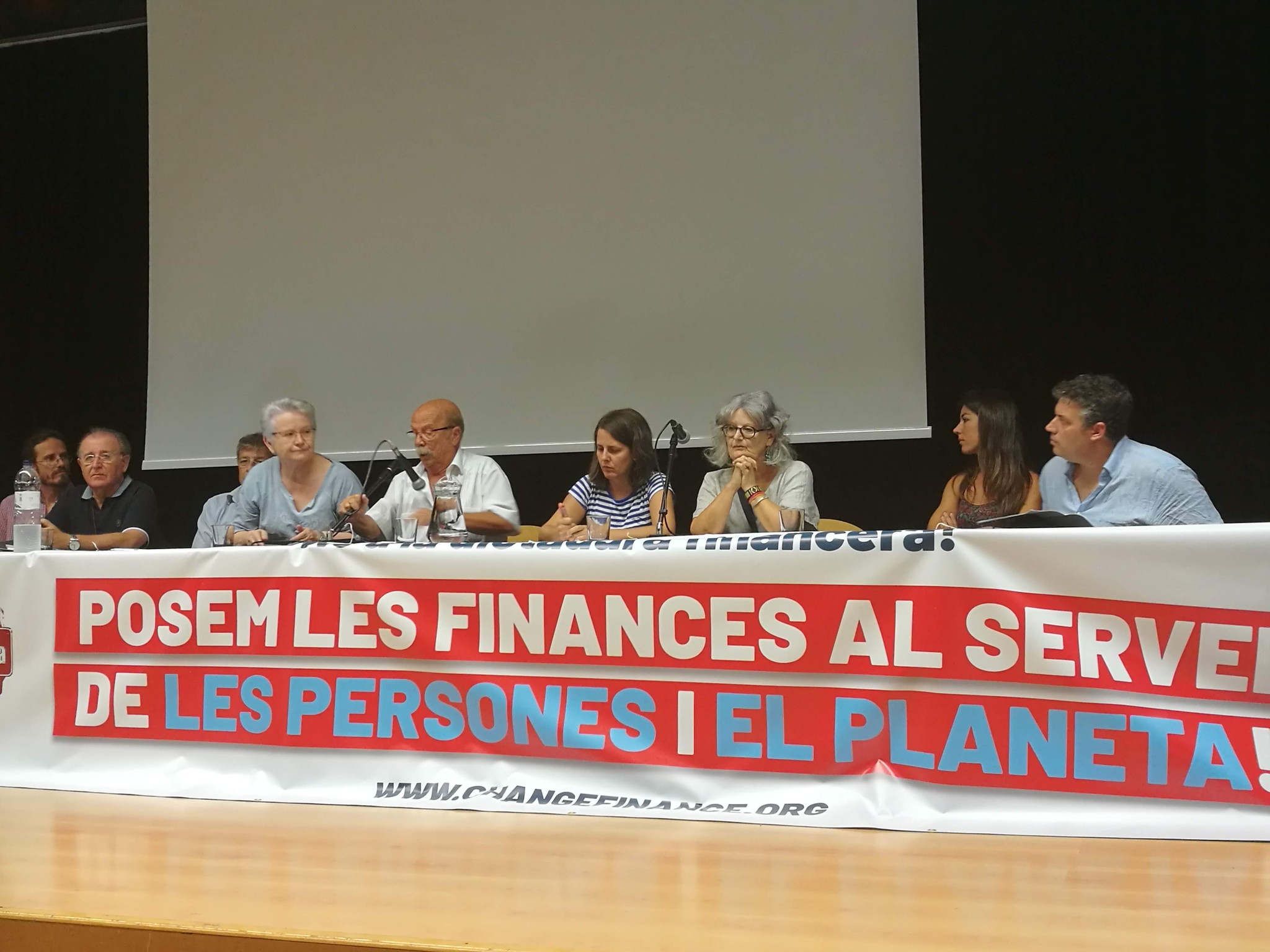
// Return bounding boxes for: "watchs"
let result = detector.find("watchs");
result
[67,535,81,550]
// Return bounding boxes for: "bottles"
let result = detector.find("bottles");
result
[13,460,41,553]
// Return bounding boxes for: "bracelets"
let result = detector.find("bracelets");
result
[320,531,326,541]
[745,485,769,508]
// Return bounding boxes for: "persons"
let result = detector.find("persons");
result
[0,427,158,553]
[690,390,820,535]
[337,399,521,543]
[191,398,369,548]
[538,407,676,541]
[927,389,1041,530]
[1039,374,1224,528]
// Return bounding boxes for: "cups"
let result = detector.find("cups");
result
[779,509,805,532]
[586,515,611,540]
[211,524,234,547]
[41,528,54,550]
[394,518,419,543]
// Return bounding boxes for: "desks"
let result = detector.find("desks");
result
[0,522,1270,816]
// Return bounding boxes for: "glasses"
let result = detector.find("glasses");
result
[721,424,770,439]
[271,427,316,438]
[406,426,454,442]
[237,456,266,468]
[84,453,115,464]
[36,456,74,465]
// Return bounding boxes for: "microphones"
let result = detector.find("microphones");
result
[669,419,690,444]
[388,441,426,491]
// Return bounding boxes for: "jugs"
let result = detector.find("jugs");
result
[426,476,468,543]
